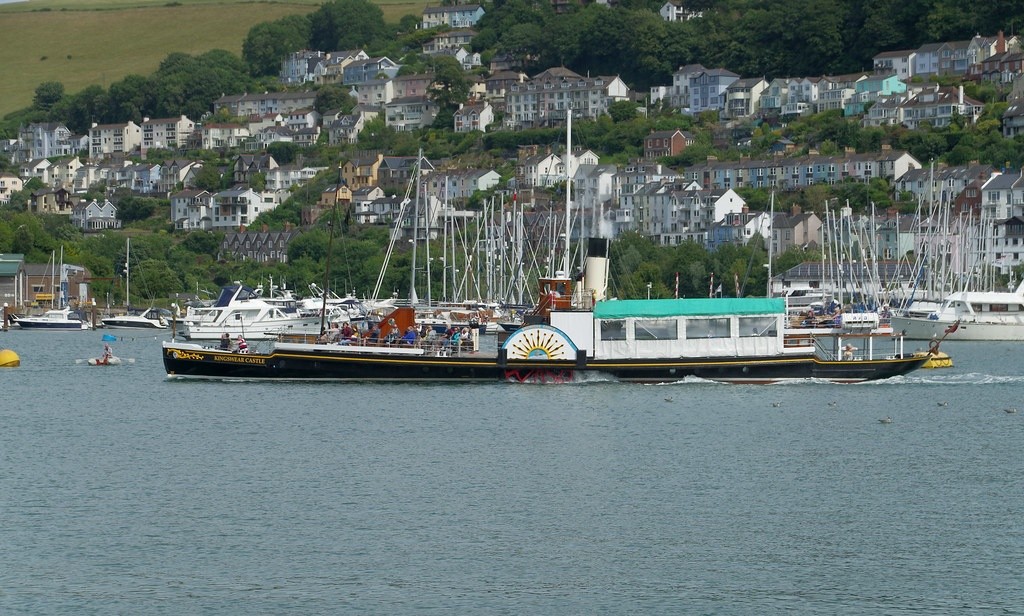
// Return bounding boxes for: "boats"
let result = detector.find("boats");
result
[103,310,168,328]
[15,307,81,328]
[892,290,1020,342]
[162,110,961,380]
[181,287,402,340]
[89,355,121,364]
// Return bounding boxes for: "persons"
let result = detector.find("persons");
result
[316,321,469,357]
[101,343,112,357]
[801,310,818,328]
[219,333,248,354]
[880,304,897,318]
[844,343,853,362]
